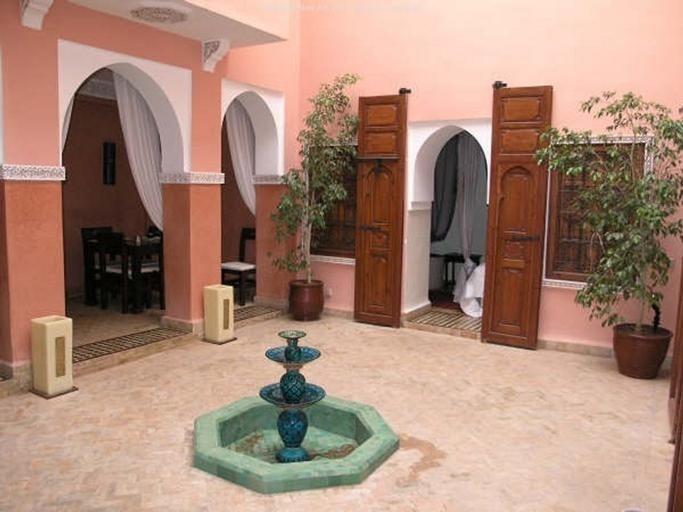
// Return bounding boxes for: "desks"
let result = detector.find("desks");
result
[443,251,483,287]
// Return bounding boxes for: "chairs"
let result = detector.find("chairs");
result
[220,227,256,306]
[80,226,164,315]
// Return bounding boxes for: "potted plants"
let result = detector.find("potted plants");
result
[267,72,359,322]
[531,89,680,380]
